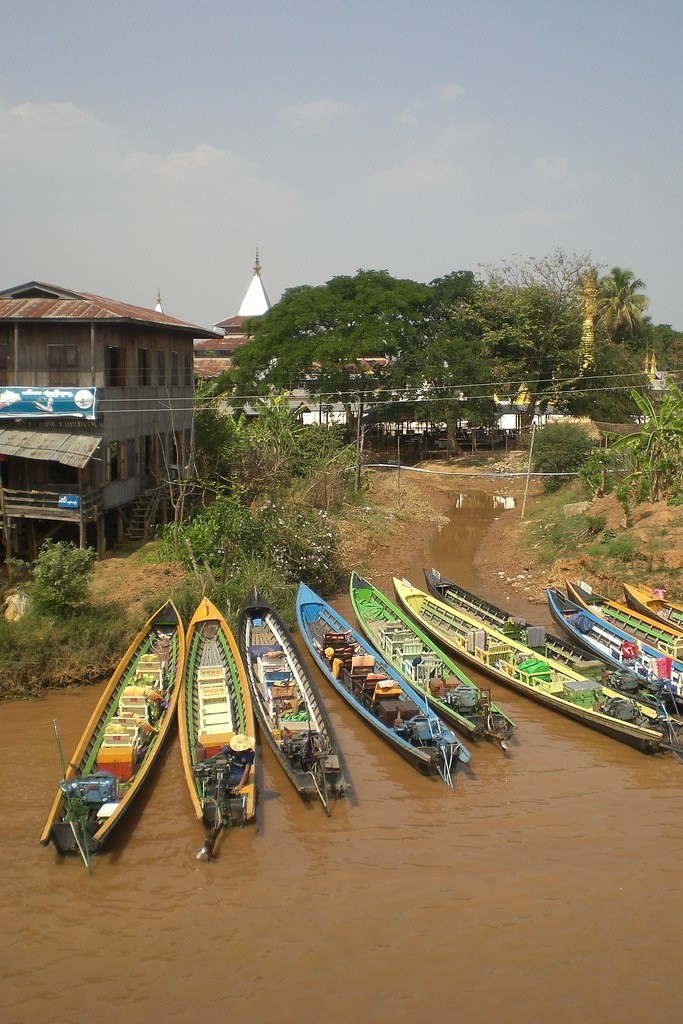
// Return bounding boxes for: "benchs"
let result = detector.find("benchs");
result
[321,619,444,717]
[96,653,163,764]
[657,639,683,659]
[249,652,311,731]
[196,665,234,735]
[455,629,574,697]
[498,617,547,659]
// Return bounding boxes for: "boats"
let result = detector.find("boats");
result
[237,585,358,812]
[290,579,474,784]
[347,569,520,749]
[187,593,257,862]
[39,594,187,860]
[392,576,682,767]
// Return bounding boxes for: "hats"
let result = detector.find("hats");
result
[230,734,255,751]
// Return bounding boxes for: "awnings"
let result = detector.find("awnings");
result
[0,425,104,469]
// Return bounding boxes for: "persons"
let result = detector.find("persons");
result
[221,734,255,794]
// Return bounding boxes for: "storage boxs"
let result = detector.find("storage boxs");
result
[429,678,463,698]
[571,660,606,682]
[97,747,134,783]
[198,733,235,759]
[378,701,420,728]
[563,680,603,710]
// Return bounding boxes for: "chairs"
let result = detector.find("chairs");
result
[610,640,639,664]
